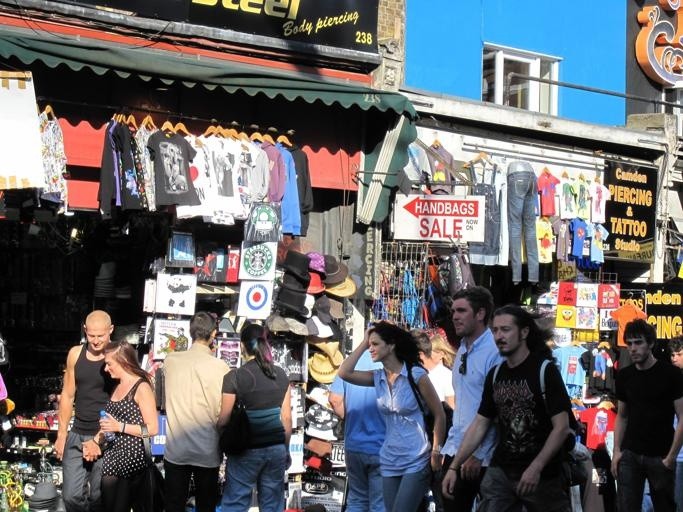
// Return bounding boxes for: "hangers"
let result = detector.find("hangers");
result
[461,146,500,173]
[553,332,615,434]
[429,128,440,148]
[36,99,299,149]
[538,160,605,229]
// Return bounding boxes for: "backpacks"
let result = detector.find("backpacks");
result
[449,252,477,299]
[492,359,598,487]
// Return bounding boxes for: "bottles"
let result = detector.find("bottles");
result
[0,488,10,512]
[100,410,115,442]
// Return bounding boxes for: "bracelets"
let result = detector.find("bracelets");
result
[93,438,99,446]
[122,423,125,433]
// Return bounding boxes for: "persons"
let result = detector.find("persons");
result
[54,310,114,512]
[217,324,291,512]
[163,312,232,512]
[81,341,159,512]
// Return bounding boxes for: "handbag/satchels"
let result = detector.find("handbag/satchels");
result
[426,248,453,325]
[421,400,456,449]
[218,369,251,460]
[128,424,167,512]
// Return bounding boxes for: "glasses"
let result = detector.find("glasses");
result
[459,351,468,375]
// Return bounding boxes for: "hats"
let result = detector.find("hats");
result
[304,456,333,477]
[306,387,334,412]
[303,438,332,457]
[308,352,339,384]
[306,425,338,441]
[28,482,61,512]
[264,247,361,340]
[305,403,339,431]
[308,336,343,366]
